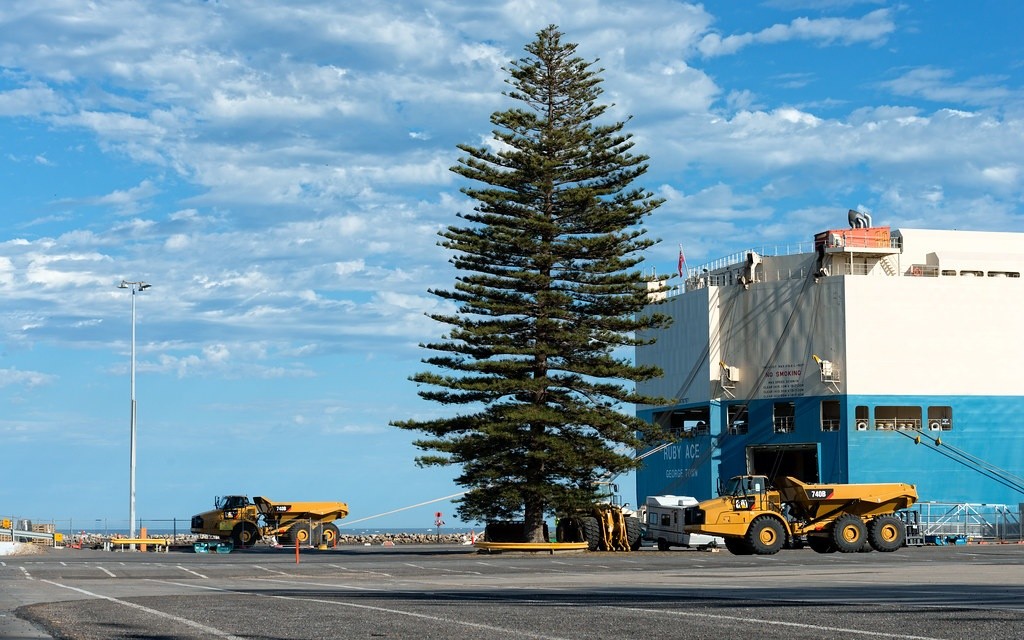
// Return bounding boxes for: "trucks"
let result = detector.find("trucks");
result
[637,495,726,551]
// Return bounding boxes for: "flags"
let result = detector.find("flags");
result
[678,251,685,278]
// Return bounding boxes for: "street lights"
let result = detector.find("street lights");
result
[116,279,151,551]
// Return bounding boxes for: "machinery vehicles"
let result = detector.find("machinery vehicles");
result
[556,479,641,553]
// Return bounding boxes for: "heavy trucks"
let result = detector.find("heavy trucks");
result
[190,496,349,550]
[683,474,918,555]
